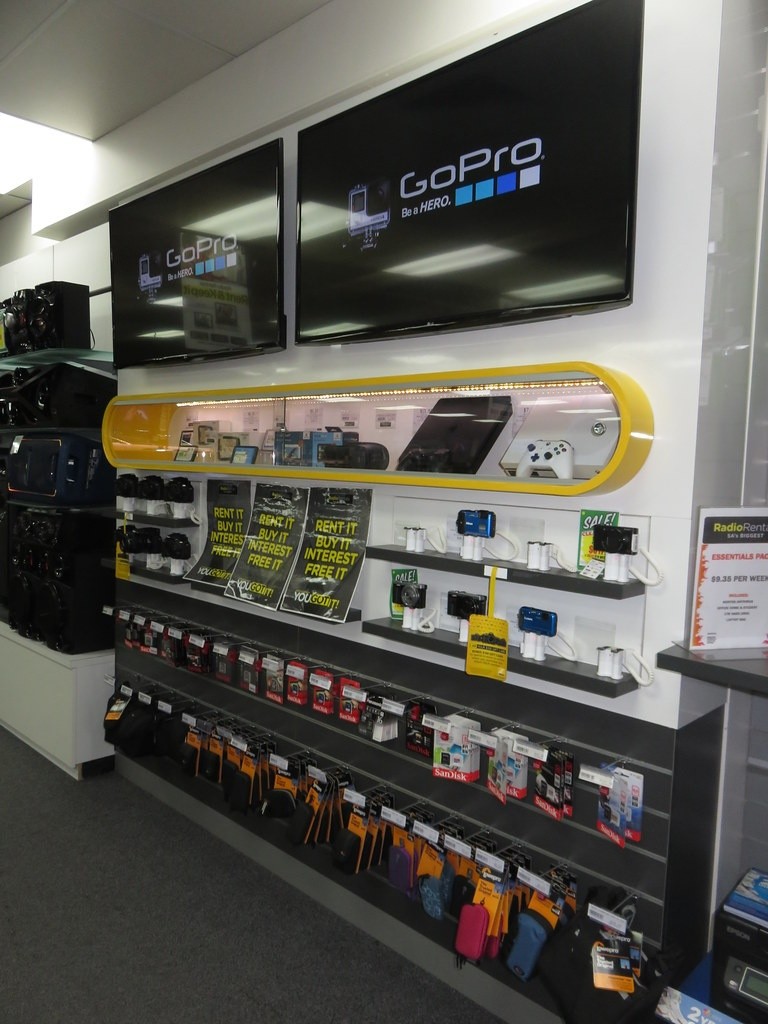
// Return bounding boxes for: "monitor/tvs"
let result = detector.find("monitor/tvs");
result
[108,0,645,367]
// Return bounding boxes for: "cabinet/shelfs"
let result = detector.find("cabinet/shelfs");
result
[96,360,768,1024]
[0,347,117,780]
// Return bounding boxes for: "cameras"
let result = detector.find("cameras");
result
[455,511,496,538]
[392,583,558,637]
[592,525,639,555]
[115,473,195,560]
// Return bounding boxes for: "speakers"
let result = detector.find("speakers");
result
[9,507,115,655]
[1,281,91,355]
[6,432,120,508]
[0,362,118,428]
